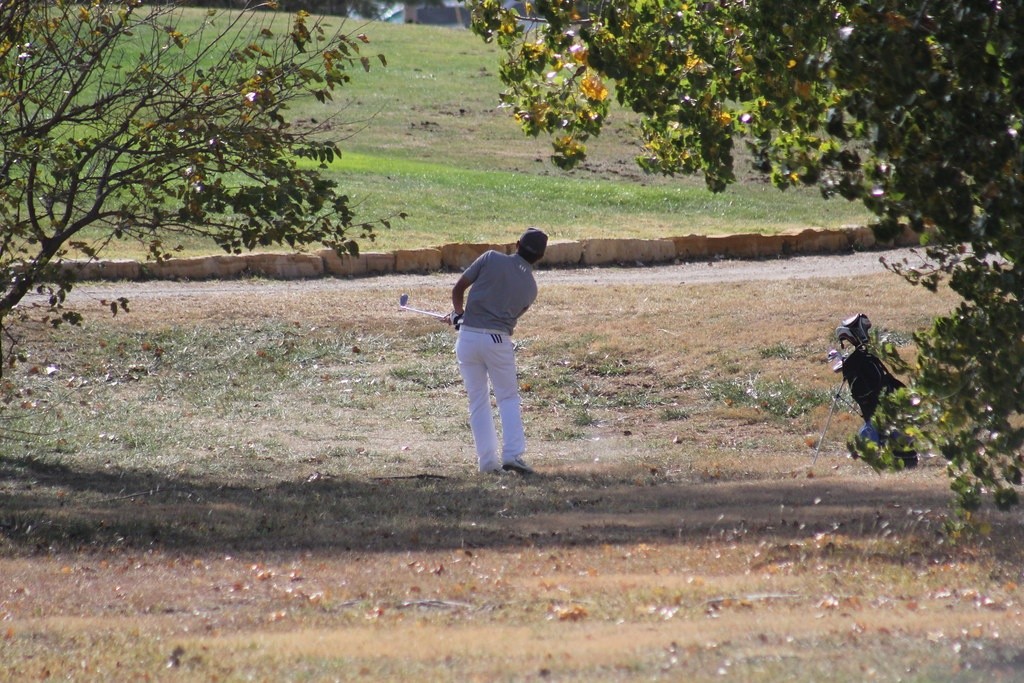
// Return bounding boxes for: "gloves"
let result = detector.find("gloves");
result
[450,310,463,330]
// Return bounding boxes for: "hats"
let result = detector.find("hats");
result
[519,227,548,256]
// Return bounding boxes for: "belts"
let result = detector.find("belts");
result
[460,324,507,335]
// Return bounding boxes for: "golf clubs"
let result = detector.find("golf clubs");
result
[824,313,871,362]
[398,293,464,325]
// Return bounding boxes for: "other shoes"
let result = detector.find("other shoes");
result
[502,457,533,474]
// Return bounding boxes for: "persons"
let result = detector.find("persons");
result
[441,227,548,474]
